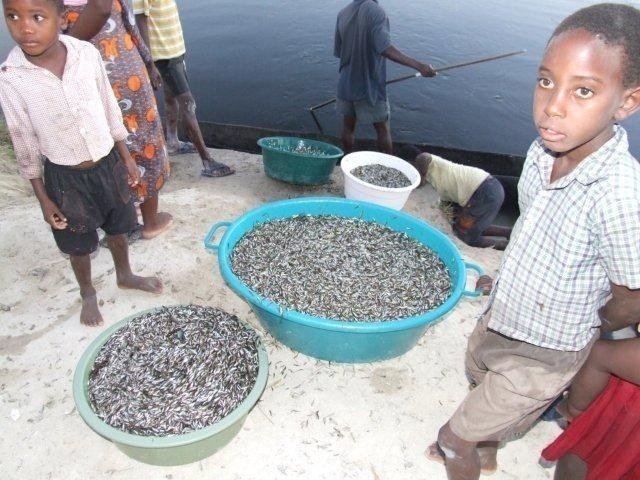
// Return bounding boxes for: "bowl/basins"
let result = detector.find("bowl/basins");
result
[201,193,490,363]
[340,149,422,213]
[72,305,271,467]
[256,135,344,188]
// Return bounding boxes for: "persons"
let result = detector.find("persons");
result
[333,0,436,157]
[62,1,174,243]
[397,140,515,251]
[433,5,640,480]
[0,1,165,328]
[130,1,238,179]
[541,333,640,480]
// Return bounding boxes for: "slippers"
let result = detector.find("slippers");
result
[168,140,199,155]
[201,162,235,177]
